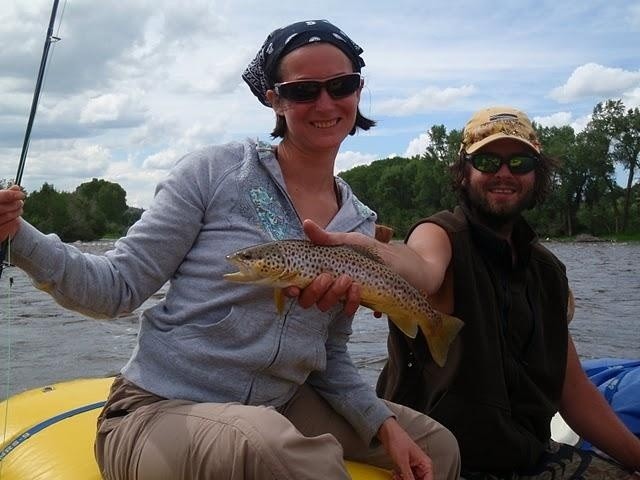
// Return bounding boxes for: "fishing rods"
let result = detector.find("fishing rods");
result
[1,6,61,283]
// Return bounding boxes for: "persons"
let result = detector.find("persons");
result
[300,106,640,478]
[0,18,463,478]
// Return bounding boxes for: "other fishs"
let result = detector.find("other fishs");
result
[222,239,466,370]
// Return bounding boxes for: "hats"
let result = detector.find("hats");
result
[460,107,542,158]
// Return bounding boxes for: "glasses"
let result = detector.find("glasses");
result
[470,151,536,174]
[272,72,361,102]
